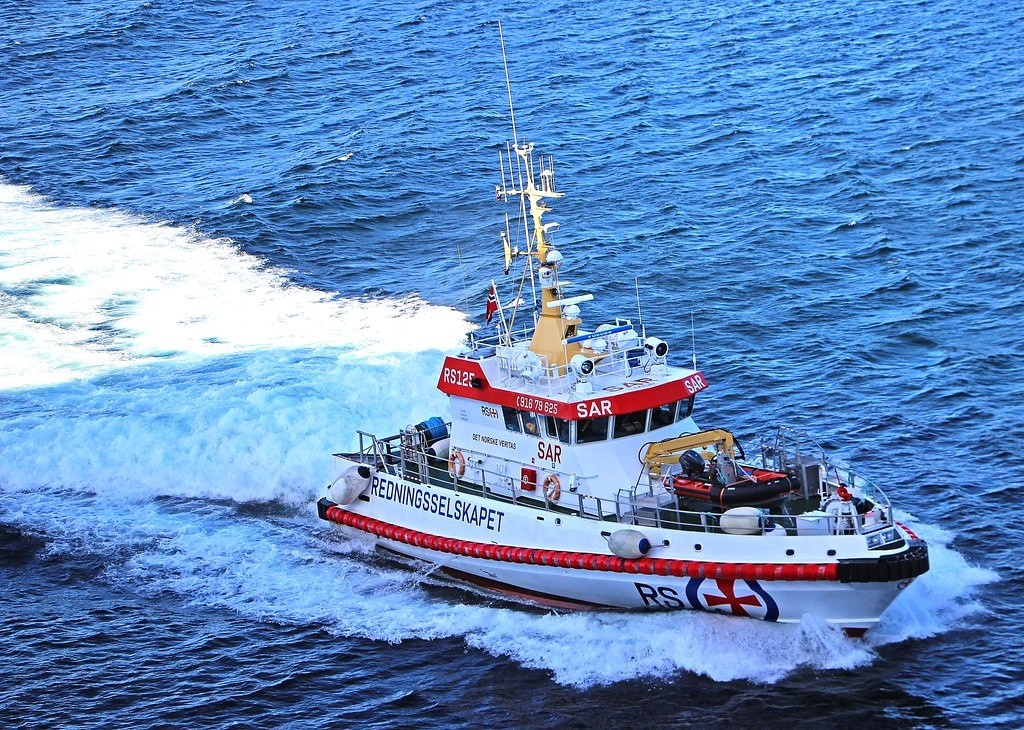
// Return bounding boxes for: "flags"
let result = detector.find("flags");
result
[485,287,498,326]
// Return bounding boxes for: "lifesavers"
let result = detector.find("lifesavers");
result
[542,474,561,503]
[447,451,466,480]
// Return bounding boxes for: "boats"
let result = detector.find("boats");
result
[317,28,930,638]
